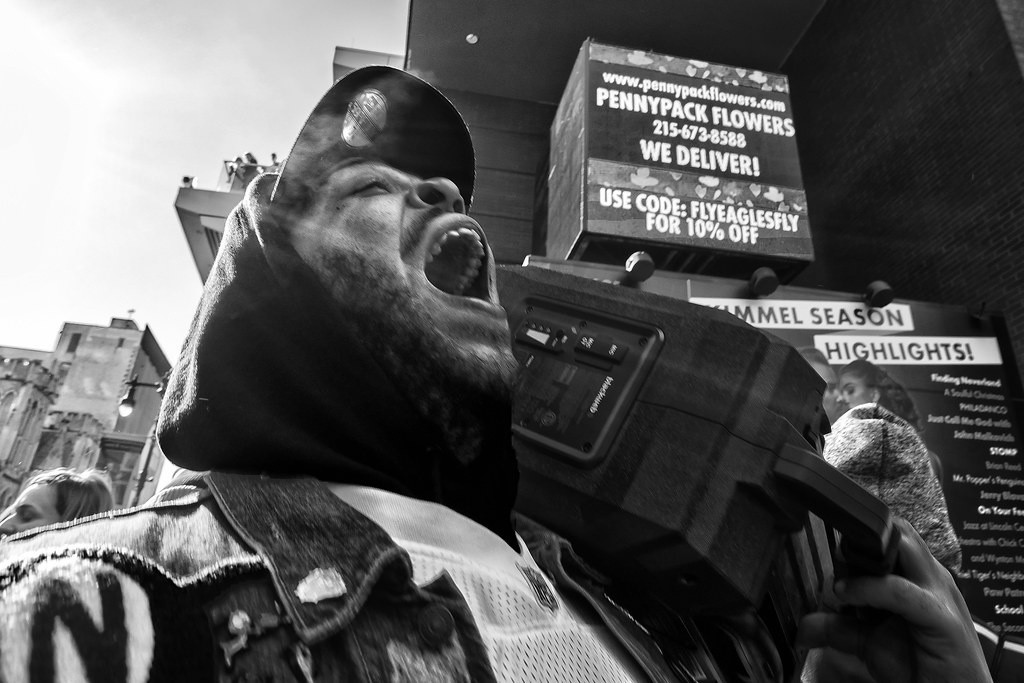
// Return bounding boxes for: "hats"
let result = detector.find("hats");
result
[270,65,477,215]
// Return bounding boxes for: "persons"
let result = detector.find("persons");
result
[0,467,115,535]
[839,356,943,485]
[1,62,998,683]
[798,347,843,424]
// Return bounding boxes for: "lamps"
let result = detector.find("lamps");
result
[751,267,778,294]
[864,281,894,308]
[625,251,655,280]
